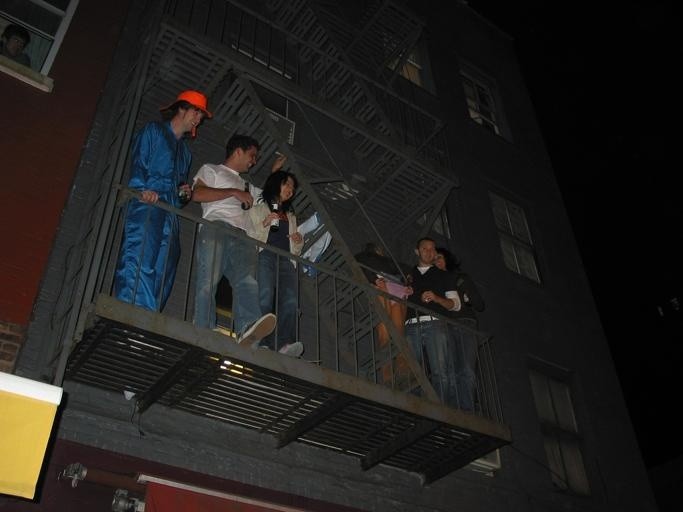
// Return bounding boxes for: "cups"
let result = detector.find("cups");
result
[177,181,188,204]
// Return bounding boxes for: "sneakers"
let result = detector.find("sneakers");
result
[238,313,277,346]
[278,342,303,356]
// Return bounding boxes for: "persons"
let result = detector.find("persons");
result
[189,132,288,348]
[114,90,213,312]
[436,244,487,411]
[250,170,305,359]
[404,236,462,409]
[0,23,31,68]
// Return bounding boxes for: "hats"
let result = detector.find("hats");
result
[159,90,214,122]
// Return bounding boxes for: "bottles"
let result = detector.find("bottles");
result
[269,203,280,233]
[241,181,251,210]
